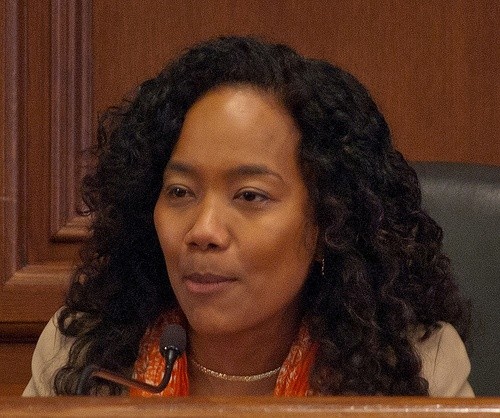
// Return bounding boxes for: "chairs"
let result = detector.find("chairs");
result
[401,161,499,399]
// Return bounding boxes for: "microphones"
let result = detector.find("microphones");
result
[76,324,186,396]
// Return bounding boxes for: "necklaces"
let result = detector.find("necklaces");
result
[187,352,283,382]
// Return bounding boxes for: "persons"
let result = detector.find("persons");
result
[21,33,477,398]
